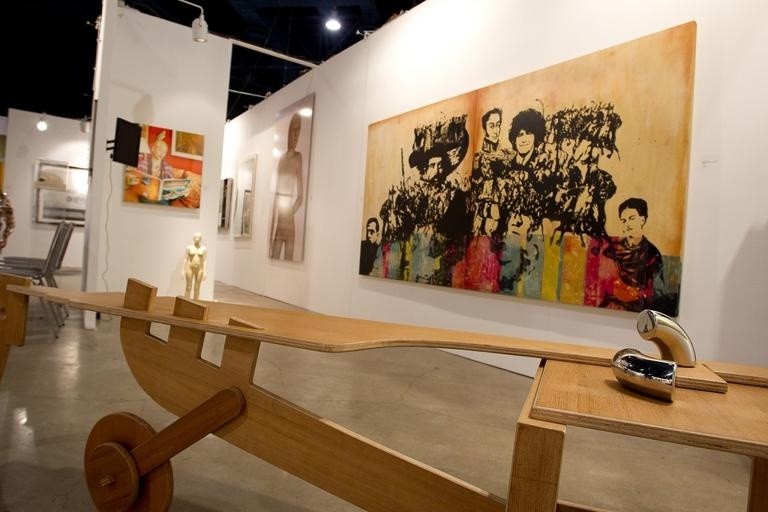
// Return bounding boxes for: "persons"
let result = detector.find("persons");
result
[181,234,207,300]
[270,114,303,260]
[126,140,190,205]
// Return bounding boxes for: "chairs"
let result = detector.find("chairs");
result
[0,221,76,339]
